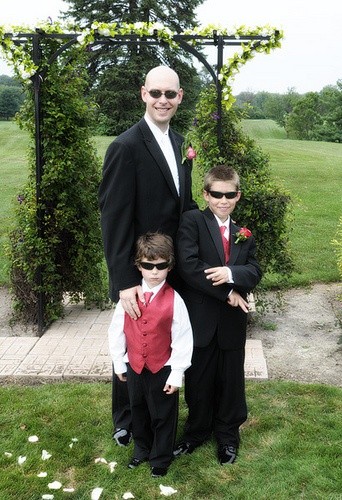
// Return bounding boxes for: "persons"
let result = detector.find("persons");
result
[108,233,193,477]
[97,65,198,445]
[173,164,262,465]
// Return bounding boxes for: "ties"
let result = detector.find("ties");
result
[220,226,230,266]
[144,292,153,307]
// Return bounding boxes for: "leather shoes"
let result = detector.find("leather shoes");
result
[173,440,195,458]
[151,466,167,477]
[218,443,237,466]
[128,458,143,468]
[113,427,132,447]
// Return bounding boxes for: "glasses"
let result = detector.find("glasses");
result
[145,87,180,99]
[207,190,238,199]
[140,262,169,270]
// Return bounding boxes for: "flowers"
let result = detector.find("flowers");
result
[234,224,253,244]
[180,139,198,166]
